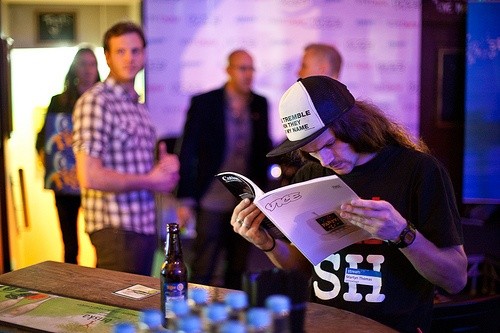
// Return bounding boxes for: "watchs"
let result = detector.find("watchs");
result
[384,218,416,249]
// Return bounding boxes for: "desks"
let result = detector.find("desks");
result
[0,260,400,333]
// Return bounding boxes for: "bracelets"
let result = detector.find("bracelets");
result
[262,234,276,252]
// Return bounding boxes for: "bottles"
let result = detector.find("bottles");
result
[160,223,188,328]
[114,289,291,332]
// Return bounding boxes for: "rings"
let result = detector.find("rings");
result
[235,217,243,225]
[242,223,251,229]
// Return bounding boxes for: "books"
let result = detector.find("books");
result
[215,171,376,266]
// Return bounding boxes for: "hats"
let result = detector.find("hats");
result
[265,75,355,158]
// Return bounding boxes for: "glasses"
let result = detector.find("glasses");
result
[231,63,256,72]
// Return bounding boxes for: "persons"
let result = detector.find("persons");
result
[71,21,180,277]
[298,44,342,81]
[173,51,274,291]
[230,75,467,333]
[36,48,102,264]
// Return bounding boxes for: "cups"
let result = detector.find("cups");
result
[243,270,308,333]
